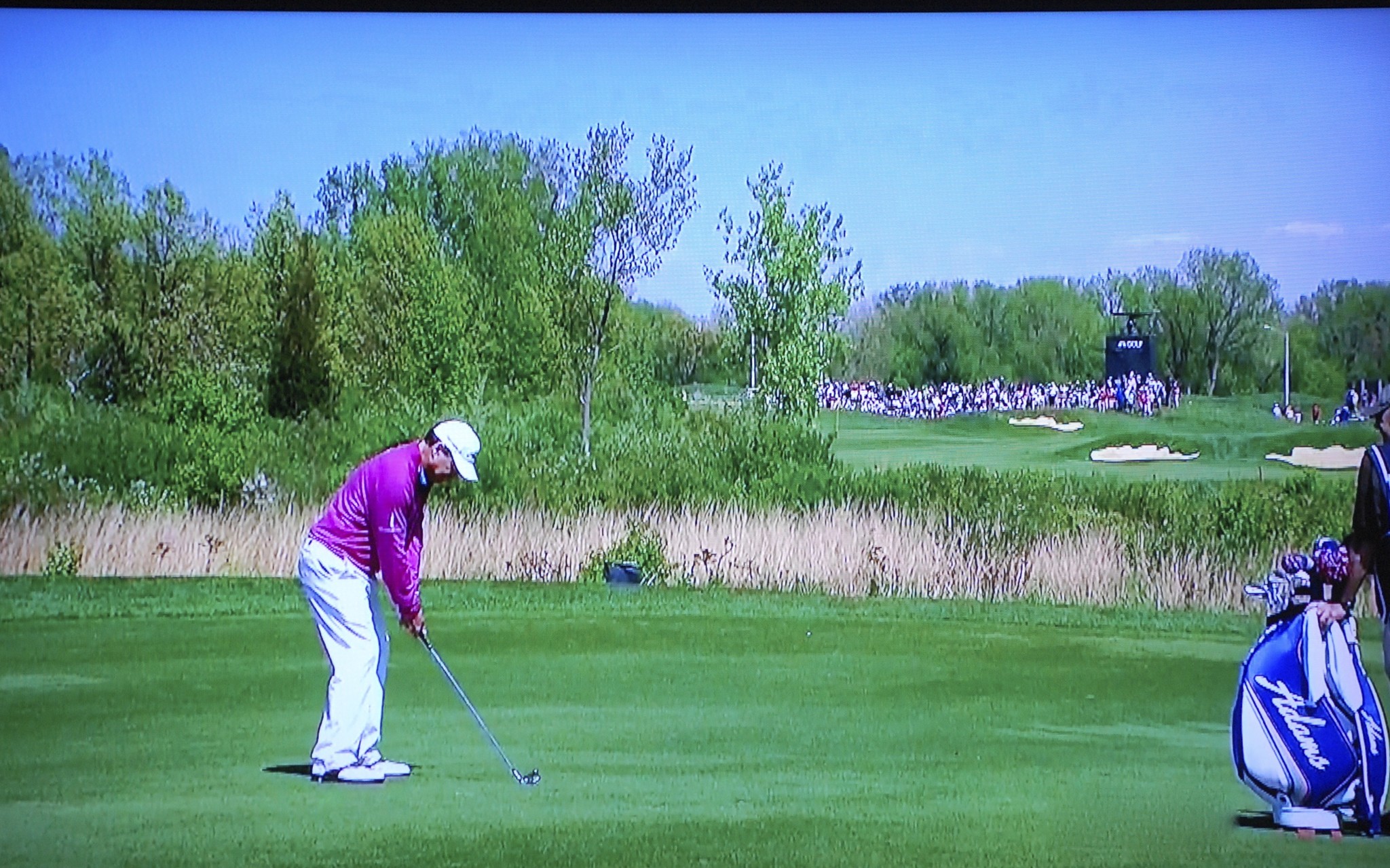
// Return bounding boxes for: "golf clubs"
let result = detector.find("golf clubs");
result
[1243,537,1351,623]
[416,627,541,786]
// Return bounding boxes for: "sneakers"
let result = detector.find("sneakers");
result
[312,762,385,783]
[366,758,411,776]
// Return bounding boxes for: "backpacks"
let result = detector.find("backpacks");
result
[1233,545,1387,837]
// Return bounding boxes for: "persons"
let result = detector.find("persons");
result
[1305,383,1390,679]
[763,368,1181,417]
[1271,386,1379,427]
[299,418,481,784]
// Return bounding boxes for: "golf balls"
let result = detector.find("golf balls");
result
[806,632,812,635]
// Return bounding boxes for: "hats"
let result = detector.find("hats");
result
[434,419,481,482]
[1362,383,1390,417]
